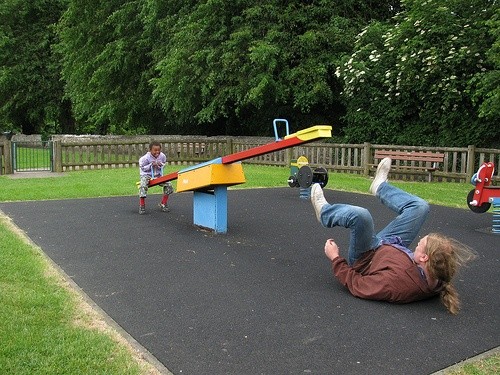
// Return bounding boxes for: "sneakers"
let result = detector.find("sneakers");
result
[369,158,391,194]
[158,202,170,212]
[139,205,145,215]
[311,183,329,222]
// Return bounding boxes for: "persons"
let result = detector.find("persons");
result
[309,156,463,315]
[137,141,173,215]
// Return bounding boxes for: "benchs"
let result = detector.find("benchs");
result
[367,148,445,187]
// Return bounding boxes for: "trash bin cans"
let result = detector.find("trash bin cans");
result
[291,156,308,176]
